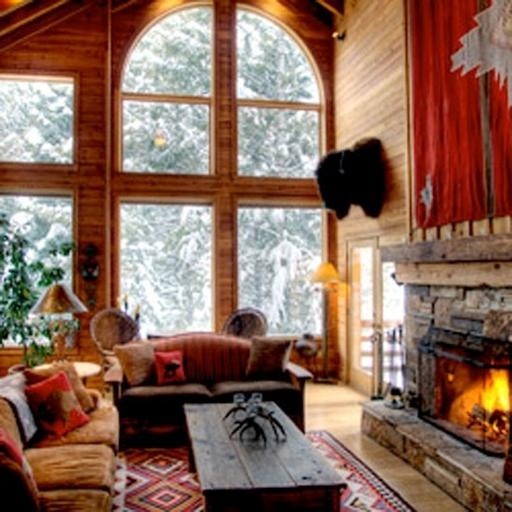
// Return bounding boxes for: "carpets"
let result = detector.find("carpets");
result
[113,430,417,512]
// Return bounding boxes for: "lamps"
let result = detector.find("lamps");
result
[308,264,346,383]
[28,285,89,359]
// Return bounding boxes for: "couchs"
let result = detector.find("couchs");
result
[102,332,313,447]
[0,371,119,511]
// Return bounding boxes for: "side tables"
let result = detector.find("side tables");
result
[28,361,101,388]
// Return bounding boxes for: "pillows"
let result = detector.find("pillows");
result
[112,342,185,387]
[24,358,96,440]
[242,335,299,380]
